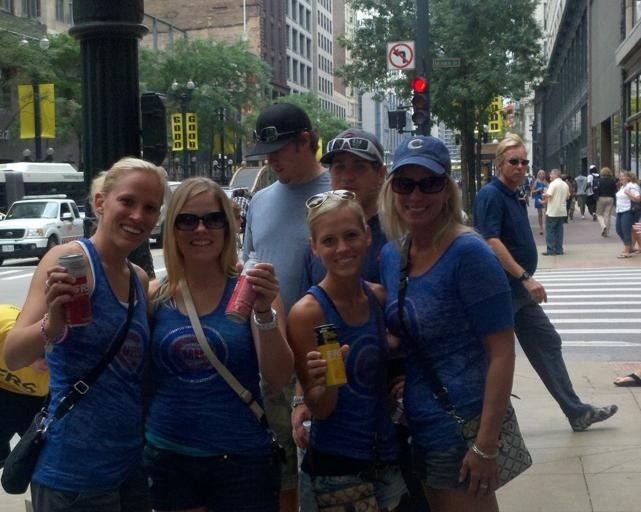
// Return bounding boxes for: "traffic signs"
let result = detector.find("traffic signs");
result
[430,58,461,67]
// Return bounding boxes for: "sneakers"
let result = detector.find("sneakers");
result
[568,404,617,432]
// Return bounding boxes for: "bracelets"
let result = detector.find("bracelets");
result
[366,137,515,511]
[253,308,281,330]
[252,306,274,316]
[137,174,295,512]
[473,441,502,463]
[38,310,71,347]
[292,395,306,405]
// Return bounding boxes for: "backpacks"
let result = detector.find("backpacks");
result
[590,172,600,193]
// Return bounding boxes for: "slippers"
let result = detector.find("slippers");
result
[614,374,640,388]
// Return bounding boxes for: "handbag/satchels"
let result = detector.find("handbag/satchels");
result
[462,407,531,490]
[1,408,52,494]
[271,442,285,466]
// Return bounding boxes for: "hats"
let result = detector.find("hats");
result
[319,129,384,165]
[245,101,311,155]
[387,136,452,177]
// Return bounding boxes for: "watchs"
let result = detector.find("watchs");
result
[516,269,529,284]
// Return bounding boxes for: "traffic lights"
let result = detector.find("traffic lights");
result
[410,76,430,126]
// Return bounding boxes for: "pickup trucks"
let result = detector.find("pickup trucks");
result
[0,194,86,266]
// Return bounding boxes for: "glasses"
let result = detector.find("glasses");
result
[389,176,448,195]
[305,188,357,209]
[326,137,384,165]
[252,126,308,143]
[508,158,529,166]
[172,211,227,230]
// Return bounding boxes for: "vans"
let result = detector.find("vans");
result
[148,182,184,246]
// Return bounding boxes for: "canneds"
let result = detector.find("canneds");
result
[57,253,93,327]
[312,324,348,387]
[224,259,264,324]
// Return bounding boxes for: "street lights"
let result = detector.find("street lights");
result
[171,79,195,182]
[482,125,498,144]
[213,106,234,189]
[17,35,55,162]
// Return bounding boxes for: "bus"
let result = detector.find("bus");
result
[0,160,88,228]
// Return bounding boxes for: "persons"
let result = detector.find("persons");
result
[479,135,621,430]
[612,370,641,389]
[243,100,336,512]
[292,187,411,510]
[230,188,250,233]
[0,301,52,466]
[4,155,167,511]
[518,164,641,260]
[292,127,413,509]
